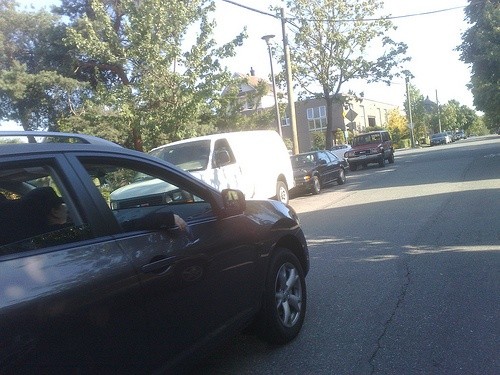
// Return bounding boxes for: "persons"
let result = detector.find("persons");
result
[19,187,188,233]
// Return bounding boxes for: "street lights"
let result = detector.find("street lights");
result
[261,33,283,139]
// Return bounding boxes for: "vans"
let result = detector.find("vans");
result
[110,131,297,212]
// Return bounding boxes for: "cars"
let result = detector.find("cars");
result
[446,131,467,142]
[289,151,350,195]
[430,131,450,145]
[330,144,353,159]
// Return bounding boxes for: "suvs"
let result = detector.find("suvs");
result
[0,131,309,375]
[348,131,394,169]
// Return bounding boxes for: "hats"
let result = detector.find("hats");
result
[22,187,67,207]
